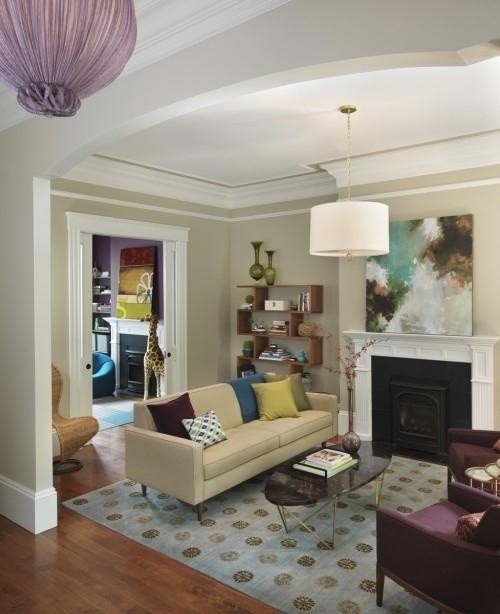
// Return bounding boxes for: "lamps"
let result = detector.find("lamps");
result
[309,104,389,262]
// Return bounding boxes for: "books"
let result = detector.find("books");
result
[94,284,106,288]
[259,344,293,360]
[101,289,110,292]
[269,320,288,336]
[298,292,311,311]
[291,448,360,478]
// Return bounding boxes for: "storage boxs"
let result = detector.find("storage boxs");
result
[265,300,292,310]
[97,334,111,353]
[92,333,97,351]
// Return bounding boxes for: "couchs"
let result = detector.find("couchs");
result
[125,373,338,521]
[93,352,116,398]
[447,427,499,488]
[376,481,500,614]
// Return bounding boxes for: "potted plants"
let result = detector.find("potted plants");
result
[241,340,254,358]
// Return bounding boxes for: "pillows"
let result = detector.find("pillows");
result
[182,409,229,448]
[146,392,197,440]
[263,372,312,411]
[451,509,488,541]
[492,438,499,453]
[250,377,302,421]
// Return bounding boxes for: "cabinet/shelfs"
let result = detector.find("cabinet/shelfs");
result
[92,277,111,333]
[236,285,324,380]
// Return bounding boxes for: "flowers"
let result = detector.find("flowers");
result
[322,335,394,389]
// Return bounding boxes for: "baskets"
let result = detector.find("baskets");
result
[297,322,318,336]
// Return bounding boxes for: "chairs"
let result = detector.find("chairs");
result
[50,362,99,473]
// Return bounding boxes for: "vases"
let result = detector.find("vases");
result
[264,251,276,286]
[249,241,265,281]
[342,389,361,453]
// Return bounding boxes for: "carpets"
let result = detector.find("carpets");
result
[62,442,451,613]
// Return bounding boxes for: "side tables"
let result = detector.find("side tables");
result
[465,459,499,497]
